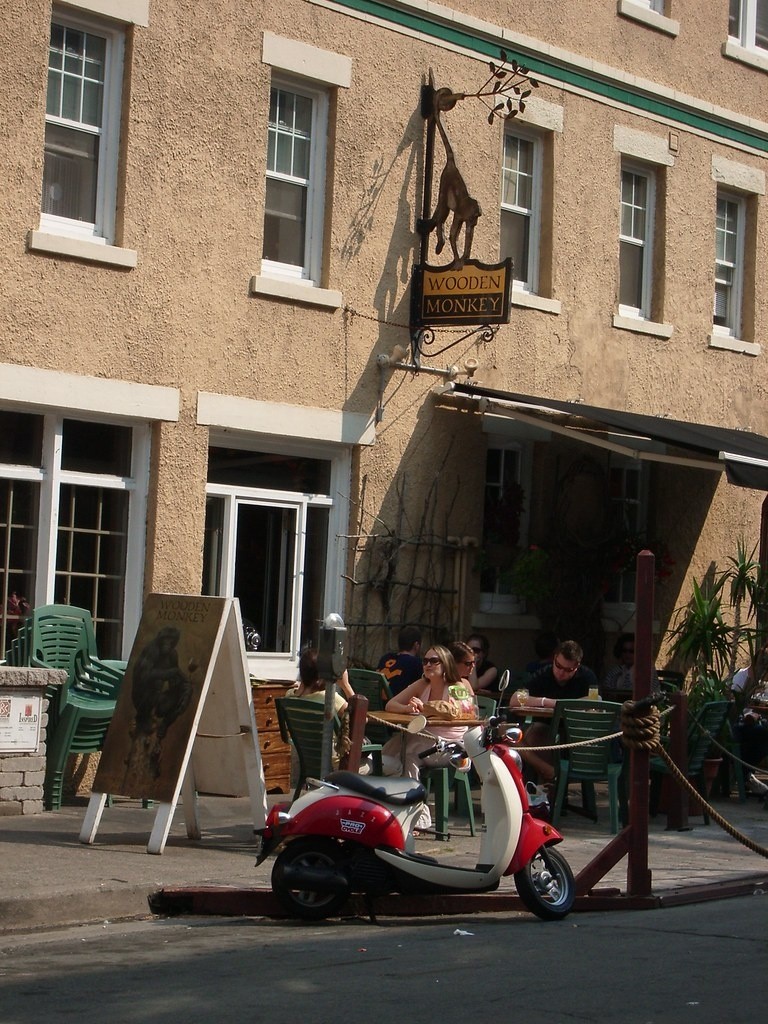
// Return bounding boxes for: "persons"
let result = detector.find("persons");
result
[712,647,768,794]
[508,640,598,760]
[604,633,664,743]
[1,589,32,651]
[285,625,555,836]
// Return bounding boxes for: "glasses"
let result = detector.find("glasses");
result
[422,658,444,665]
[554,657,579,672]
[461,660,476,666]
[470,647,485,654]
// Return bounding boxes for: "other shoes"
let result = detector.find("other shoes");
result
[748,774,768,795]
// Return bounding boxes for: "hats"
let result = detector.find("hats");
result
[422,700,458,721]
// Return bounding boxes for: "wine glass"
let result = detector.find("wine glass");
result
[516,688,529,710]
[588,687,598,711]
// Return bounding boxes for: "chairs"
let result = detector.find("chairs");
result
[418,695,497,841]
[550,698,630,837]
[648,700,736,832]
[337,667,401,747]
[655,669,685,737]
[273,696,385,802]
[0,604,155,812]
[711,716,747,804]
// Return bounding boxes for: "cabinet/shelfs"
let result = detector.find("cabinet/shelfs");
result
[251,682,300,795]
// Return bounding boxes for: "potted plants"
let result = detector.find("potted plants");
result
[654,575,751,817]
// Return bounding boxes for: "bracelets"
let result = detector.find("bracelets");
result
[541,697,546,706]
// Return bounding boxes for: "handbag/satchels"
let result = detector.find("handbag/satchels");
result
[524,781,552,815]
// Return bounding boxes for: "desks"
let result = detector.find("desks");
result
[366,710,520,842]
[471,688,515,701]
[496,705,615,824]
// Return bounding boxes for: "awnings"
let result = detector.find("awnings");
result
[451,381,768,490]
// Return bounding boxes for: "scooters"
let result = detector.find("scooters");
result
[252,669,578,926]
